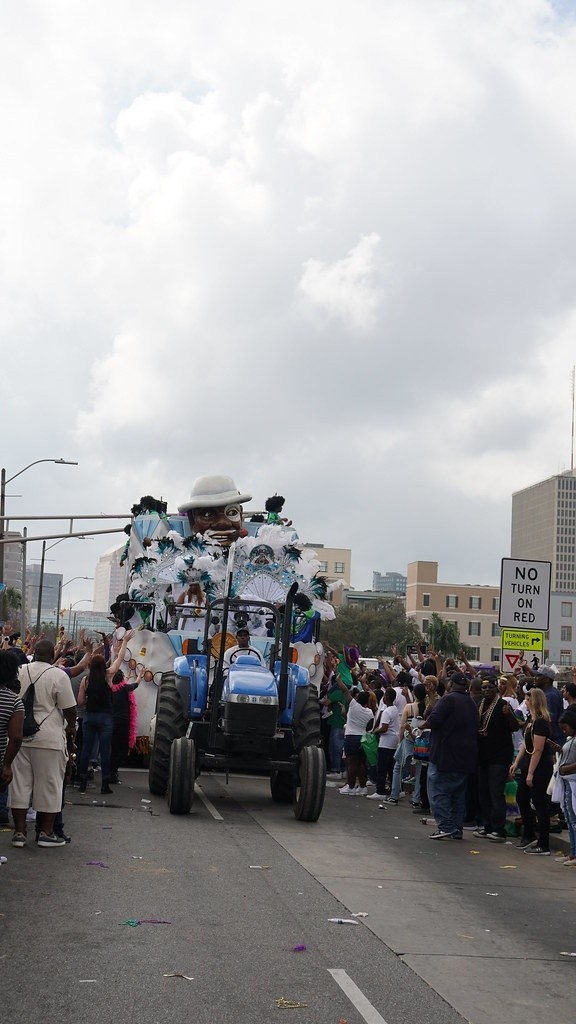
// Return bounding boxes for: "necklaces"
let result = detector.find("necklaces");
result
[478,694,500,732]
[481,710,487,715]
[524,717,536,754]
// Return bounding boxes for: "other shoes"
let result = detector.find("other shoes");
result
[322,711,332,719]
[563,858,576,866]
[516,836,538,850]
[326,767,482,839]
[473,828,488,838]
[554,855,572,862]
[55,831,71,843]
[100,788,114,794]
[524,846,551,856]
[487,831,505,840]
[78,787,86,794]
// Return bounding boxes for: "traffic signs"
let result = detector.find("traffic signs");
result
[500,628,545,675]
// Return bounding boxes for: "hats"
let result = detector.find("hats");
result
[485,665,492,669]
[451,672,467,685]
[343,645,359,668]
[529,664,555,680]
[480,675,500,693]
[237,626,249,637]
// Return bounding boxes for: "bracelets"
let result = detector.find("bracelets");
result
[126,677,129,679]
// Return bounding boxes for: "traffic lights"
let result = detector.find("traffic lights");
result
[59,626,65,639]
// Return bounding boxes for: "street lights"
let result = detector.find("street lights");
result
[0,457,80,644]
[34,535,85,637]
[55,576,95,636]
[72,610,93,640]
[67,599,91,635]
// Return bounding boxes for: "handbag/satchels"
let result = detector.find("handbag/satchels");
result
[412,730,431,761]
[546,752,562,795]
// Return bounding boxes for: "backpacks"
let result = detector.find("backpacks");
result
[18,665,56,738]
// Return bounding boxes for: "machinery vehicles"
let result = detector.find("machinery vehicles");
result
[113,570,328,822]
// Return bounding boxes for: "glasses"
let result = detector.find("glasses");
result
[482,685,498,690]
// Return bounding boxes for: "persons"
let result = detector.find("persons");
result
[223,629,265,678]
[0,624,150,869]
[120,496,167,566]
[319,641,576,867]
[273,593,315,637]
[251,495,292,526]
[177,475,252,548]
[177,583,209,631]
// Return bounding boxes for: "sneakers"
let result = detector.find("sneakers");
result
[37,832,65,846]
[11,832,26,847]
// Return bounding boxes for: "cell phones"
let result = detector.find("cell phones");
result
[546,739,561,750]
